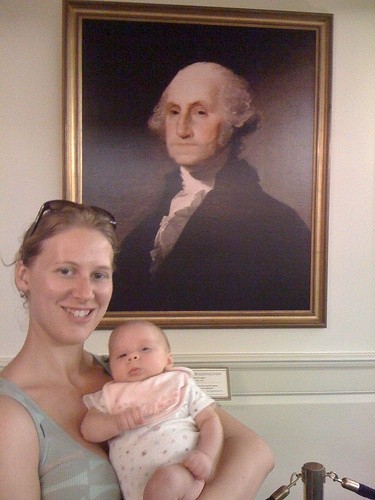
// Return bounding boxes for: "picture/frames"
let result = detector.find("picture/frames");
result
[62,0,334,331]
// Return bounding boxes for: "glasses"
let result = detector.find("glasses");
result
[29,200,116,239]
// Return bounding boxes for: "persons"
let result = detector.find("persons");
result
[0,199,275,500]
[81,318,223,500]
[107,61,312,312]
